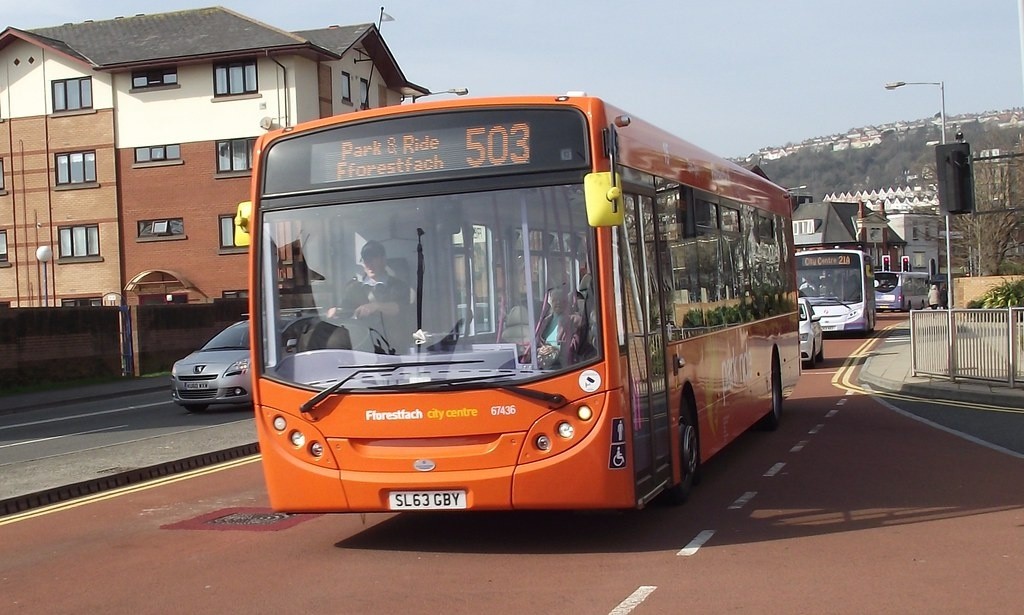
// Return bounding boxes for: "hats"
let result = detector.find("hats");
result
[359,240,385,261]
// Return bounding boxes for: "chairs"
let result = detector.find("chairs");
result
[386,259,410,285]
[494,273,594,365]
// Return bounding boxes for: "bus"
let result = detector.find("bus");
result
[874,269,930,313]
[793,246,877,339]
[234,90,802,514]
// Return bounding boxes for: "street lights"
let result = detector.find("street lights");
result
[886,80,955,384]
[37,244,53,306]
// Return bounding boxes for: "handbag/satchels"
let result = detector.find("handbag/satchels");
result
[535,342,560,369]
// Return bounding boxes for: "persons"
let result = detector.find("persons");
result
[329,241,409,335]
[940,284,948,309]
[928,285,940,310]
[534,288,583,368]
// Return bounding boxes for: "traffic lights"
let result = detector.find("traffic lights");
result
[901,255,910,272]
[881,255,891,272]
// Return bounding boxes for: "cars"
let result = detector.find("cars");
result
[798,297,823,369]
[170,314,323,413]
